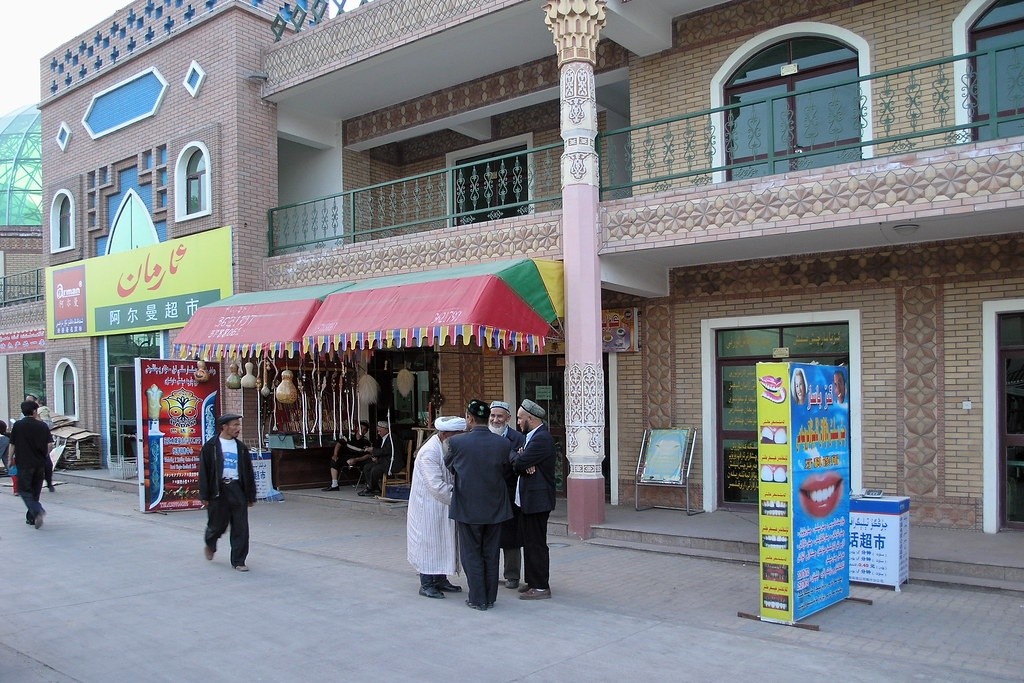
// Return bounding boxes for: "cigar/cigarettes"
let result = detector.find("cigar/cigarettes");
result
[200,504,205,509]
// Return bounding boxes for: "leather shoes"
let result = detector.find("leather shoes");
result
[467,600,494,611]
[439,580,462,593]
[419,583,444,598]
[321,485,339,491]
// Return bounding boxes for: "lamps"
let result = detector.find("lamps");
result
[892,225,920,236]
[248,73,269,83]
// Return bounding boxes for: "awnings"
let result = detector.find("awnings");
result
[171,280,357,361]
[302,257,565,354]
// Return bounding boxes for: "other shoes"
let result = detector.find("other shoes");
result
[14,493,19,496]
[232,566,248,572]
[206,545,213,560]
[505,580,519,589]
[49,486,54,492]
[35,508,46,529]
[26,520,34,525]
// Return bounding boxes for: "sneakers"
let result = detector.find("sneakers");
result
[358,487,381,496]
[518,585,551,599]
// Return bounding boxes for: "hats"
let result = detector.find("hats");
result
[520,399,545,419]
[468,399,491,418]
[377,421,388,429]
[490,401,511,413]
[435,416,467,431]
[218,414,242,425]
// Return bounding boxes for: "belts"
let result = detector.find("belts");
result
[222,477,239,484]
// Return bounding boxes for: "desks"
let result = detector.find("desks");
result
[271,445,366,490]
[248,450,284,502]
[849,496,911,593]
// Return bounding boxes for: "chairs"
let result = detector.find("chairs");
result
[338,464,364,492]
[381,439,412,498]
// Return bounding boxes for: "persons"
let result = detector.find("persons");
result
[791,367,809,405]
[406,398,557,610]
[799,472,843,517]
[0,395,55,529]
[321,420,371,492]
[833,370,845,403]
[357,421,404,496]
[199,414,257,571]
